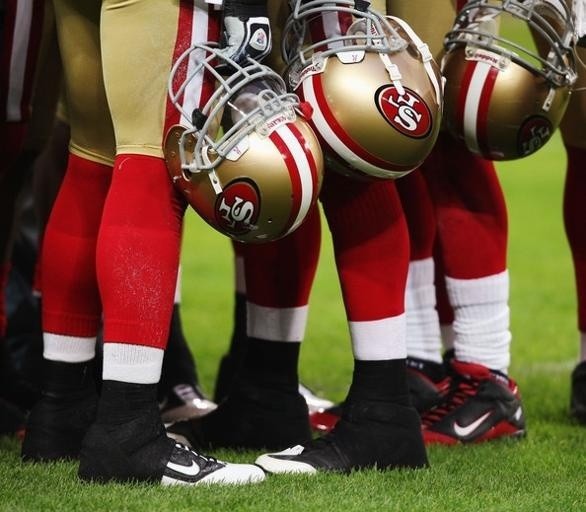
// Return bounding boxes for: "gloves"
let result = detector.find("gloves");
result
[214,0,273,76]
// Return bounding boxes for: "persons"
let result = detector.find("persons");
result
[0,1,585,489]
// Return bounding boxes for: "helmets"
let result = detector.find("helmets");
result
[164,41,324,245]
[440,0,578,161]
[281,0,447,179]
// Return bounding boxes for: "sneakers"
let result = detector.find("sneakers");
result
[0,355,525,485]
